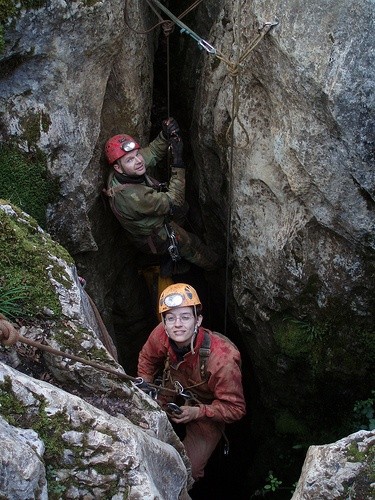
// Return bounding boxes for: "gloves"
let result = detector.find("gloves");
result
[166,136,184,165]
[161,118,180,141]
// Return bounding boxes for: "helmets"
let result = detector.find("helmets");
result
[104,134,141,166]
[158,283,202,314]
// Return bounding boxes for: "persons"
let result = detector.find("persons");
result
[104,118,218,278]
[137,282,246,490]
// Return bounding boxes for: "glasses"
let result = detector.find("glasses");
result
[163,315,195,323]
